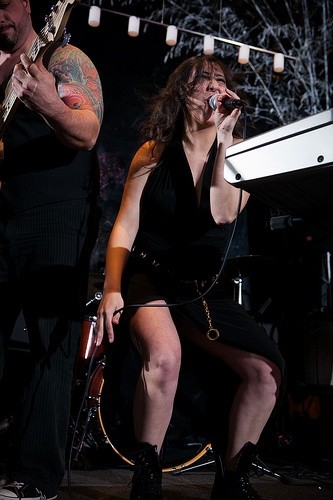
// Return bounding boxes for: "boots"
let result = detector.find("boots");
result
[205,440,261,500]
[128,442,161,500]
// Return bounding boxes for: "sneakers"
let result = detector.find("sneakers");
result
[0,481,58,500]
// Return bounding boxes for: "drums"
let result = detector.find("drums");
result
[85,363,212,473]
[77,314,104,360]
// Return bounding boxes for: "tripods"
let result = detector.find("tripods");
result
[170,274,297,485]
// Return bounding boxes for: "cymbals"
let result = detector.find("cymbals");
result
[220,255,272,278]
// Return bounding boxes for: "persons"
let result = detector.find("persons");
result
[0,0,105,500]
[95,55,280,500]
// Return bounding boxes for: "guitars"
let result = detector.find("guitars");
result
[0,0,76,191]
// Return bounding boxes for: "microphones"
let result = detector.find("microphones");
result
[208,93,245,111]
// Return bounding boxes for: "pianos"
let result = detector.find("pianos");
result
[222,106,333,194]
